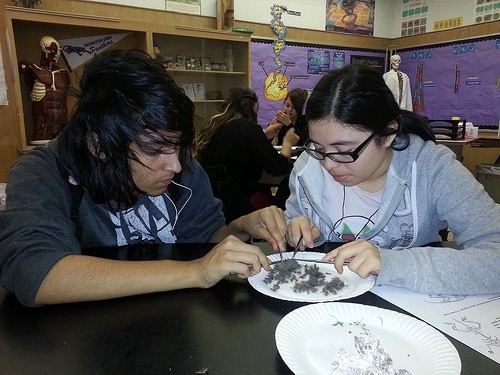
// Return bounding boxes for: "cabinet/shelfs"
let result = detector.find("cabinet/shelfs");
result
[133,31,251,159]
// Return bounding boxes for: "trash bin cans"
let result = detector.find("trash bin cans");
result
[475,164,500,203]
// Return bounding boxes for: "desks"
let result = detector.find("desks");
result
[433,139,475,162]
[0,242,500,375]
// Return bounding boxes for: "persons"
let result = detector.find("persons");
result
[266,88,309,146]
[284,63,500,295]
[0,49,272,308]
[18,35,70,140]
[195,85,300,225]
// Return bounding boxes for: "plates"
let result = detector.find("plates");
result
[274,303,462,375]
[274,145,299,150]
[246,251,375,302]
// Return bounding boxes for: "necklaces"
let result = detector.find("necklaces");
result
[328,185,379,242]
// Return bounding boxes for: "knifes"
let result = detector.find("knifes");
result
[230,260,287,274]
[291,235,303,259]
[292,258,350,265]
[278,246,283,263]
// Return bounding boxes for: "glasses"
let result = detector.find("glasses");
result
[303,129,379,163]
